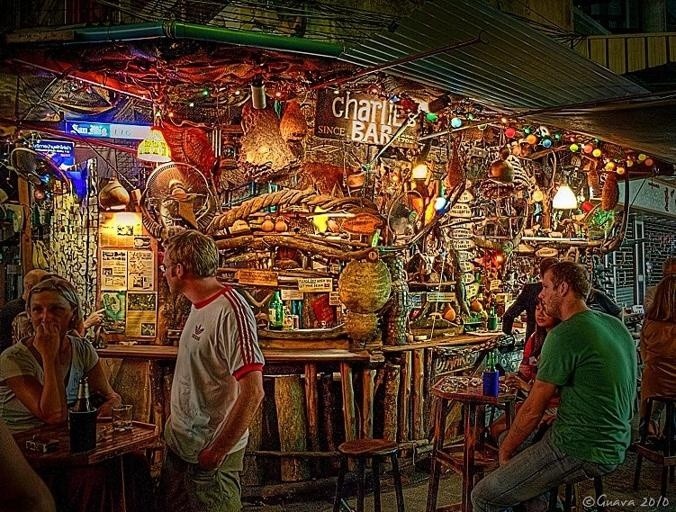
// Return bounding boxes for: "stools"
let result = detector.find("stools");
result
[548,475,605,512]
[629,395,676,501]
[333,438,405,512]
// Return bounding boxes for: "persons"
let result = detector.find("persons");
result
[641,257,676,438]
[0,268,123,508]
[152,231,265,512]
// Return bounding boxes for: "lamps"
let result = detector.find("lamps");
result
[136,116,172,163]
[553,172,577,209]
[413,163,427,179]
[250,75,267,109]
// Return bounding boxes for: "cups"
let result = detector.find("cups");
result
[111,405,133,435]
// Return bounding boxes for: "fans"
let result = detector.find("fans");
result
[145,162,210,221]
[10,148,71,195]
[384,190,425,236]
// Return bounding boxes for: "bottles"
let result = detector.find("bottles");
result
[487,306,496,330]
[483,351,499,398]
[68,376,96,455]
[267,290,282,330]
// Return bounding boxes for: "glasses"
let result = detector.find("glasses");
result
[159,263,179,272]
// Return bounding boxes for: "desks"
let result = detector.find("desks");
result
[8,416,161,511]
